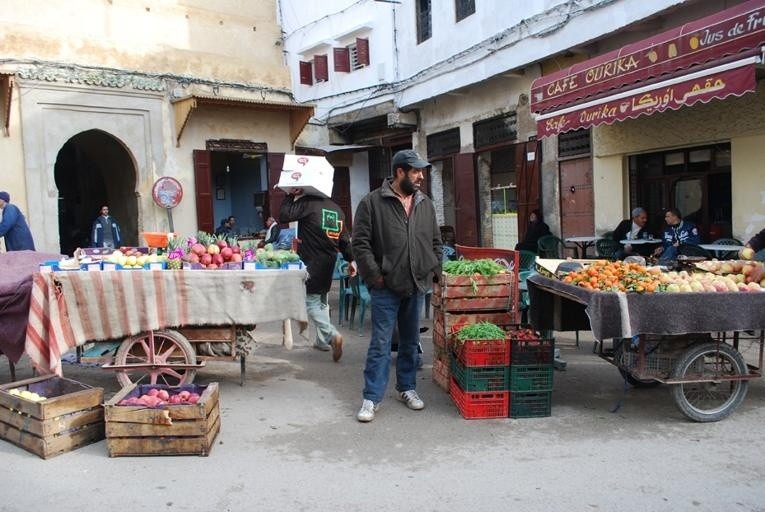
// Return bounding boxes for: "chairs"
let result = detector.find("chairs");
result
[517,231,765,347]
[332,225,458,335]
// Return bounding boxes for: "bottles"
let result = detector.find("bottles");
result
[632,334,648,353]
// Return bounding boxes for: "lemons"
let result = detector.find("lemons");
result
[7,389,48,403]
[110,250,166,269]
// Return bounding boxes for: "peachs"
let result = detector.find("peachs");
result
[661,248,765,294]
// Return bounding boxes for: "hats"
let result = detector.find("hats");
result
[0,192,9,202]
[391,149,432,172]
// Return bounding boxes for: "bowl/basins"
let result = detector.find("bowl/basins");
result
[554,261,582,276]
[141,231,176,246]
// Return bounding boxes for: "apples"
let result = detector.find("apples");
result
[181,239,243,268]
[118,388,202,408]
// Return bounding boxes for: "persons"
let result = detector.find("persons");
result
[358,149,443,422]
[515,209,549,258]
[278,187,357,362]
[738,229,765,259]
[216,219,230,241]
[258,217,280,248]
[91,205,123,249]
[0,192,35,251]
[612,207,655,260]
[227,217,237,237]
[656,207,705,265]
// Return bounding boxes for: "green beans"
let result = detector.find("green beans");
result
[459,319,507,342]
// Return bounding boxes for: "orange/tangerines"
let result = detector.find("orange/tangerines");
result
[563,260,668,293]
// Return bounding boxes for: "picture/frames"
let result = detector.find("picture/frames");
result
[216,188,225,200]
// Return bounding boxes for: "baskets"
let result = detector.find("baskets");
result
[623,351,705,378]
[450,323,555,421]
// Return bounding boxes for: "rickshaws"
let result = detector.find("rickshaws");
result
[529,255,765,423]
[1,241,303,393]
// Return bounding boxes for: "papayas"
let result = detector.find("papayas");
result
[255,242,299,265]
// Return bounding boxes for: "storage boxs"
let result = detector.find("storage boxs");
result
[103,381,220,458]
[0,374,106,459]
[277,170,334,199]
[431,268,515,393]
[282,153,335,182]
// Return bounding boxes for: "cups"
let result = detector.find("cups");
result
[649,234,653,241]
[643,231,648,240]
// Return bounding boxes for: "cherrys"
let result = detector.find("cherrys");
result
[506,327,542,347]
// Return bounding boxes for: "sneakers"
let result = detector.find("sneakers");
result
[329,334,343,362]
[356,399,380,422]
[312,340,330,351]
[395,389,424,410]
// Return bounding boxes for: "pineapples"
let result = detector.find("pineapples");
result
[166,237,182,271]
